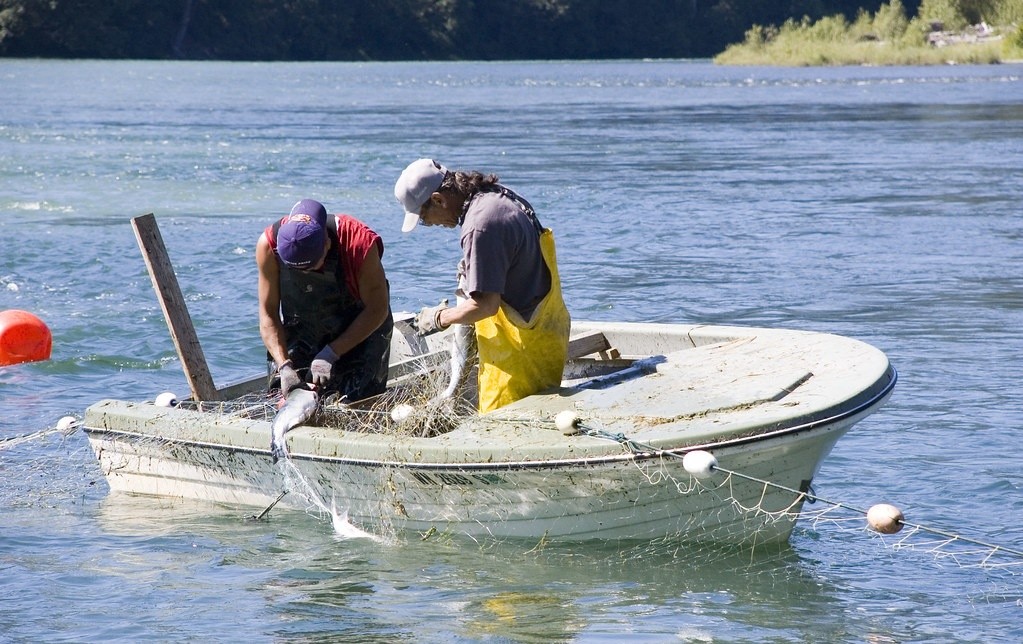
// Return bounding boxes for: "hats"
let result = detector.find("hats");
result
[276,199,327,267]
[394,159,448,232]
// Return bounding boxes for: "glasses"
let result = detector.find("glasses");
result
[419,203,433,226]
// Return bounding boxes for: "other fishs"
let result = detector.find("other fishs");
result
[429,274,473,411]
[270,382,318,461]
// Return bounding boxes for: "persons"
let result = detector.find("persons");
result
[256,198,394,403]
[394,159,571,413]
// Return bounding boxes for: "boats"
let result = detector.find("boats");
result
[80,306,898,546]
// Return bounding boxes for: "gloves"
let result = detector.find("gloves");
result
[413,299,451,339]
[305,345,340,389]
[278,359,301,399]
